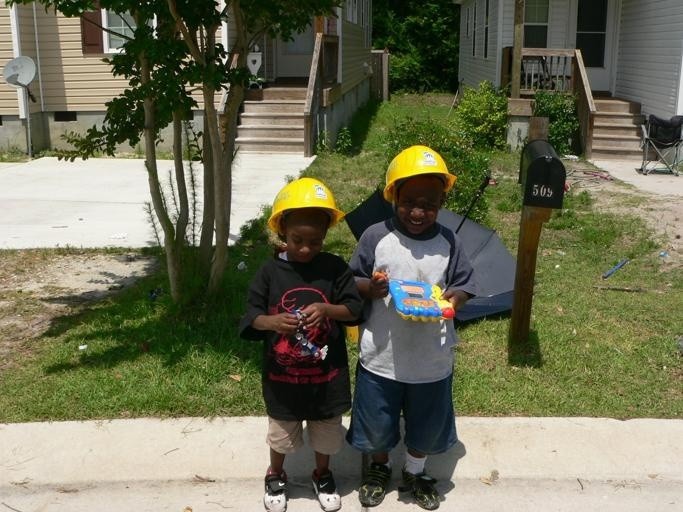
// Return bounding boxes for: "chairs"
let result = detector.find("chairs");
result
[640,114,683,175]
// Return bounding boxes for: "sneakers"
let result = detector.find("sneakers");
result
[311,468,341,512]
[263,467,288,512]
[398,464,440,510]
[358,461,392,506]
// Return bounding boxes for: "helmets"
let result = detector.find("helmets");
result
[382,144,458,204]
[268,178,346,235]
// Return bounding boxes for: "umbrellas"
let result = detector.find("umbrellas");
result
[342,174,515,323]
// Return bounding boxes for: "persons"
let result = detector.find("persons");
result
[238,176,363,512]
[346,144,477,511]
